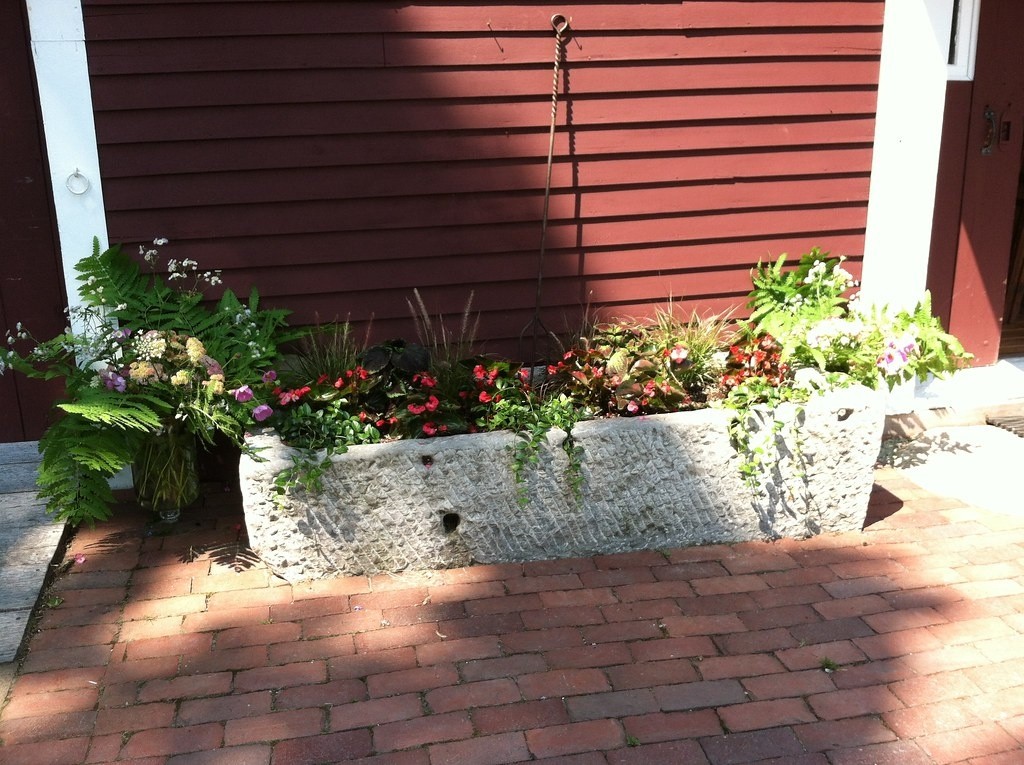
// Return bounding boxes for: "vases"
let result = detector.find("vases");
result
[131,414,200,522]
[240,368,886,585]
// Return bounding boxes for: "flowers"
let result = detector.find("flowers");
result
[272,248,973,514]
[0,237,353,530]
